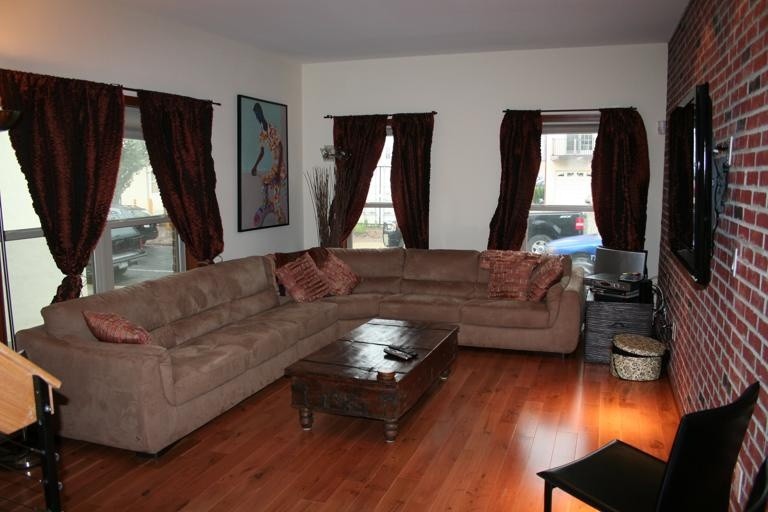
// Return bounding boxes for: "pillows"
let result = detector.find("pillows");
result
[274,251,331,304]
[527,256,563,302]
[487,250,541,302]
[319,253,363,296]
[82,309,153,344]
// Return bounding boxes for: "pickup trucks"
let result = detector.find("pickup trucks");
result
[383,201,587,254]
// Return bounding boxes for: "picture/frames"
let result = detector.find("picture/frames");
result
[237,95,289,232]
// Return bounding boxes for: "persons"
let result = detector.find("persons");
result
[251,102,288,227]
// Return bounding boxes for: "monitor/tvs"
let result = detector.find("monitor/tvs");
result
[665,81,724,286]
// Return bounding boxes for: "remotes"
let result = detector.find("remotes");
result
[390,343,417,356]
[383,346,411,360]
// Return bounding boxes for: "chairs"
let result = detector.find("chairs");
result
[536,382,760,512]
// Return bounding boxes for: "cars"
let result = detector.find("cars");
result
[108,204,159,241]
[541,233,602,262]
[82,202,146,281]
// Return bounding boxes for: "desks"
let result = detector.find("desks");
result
[584,289,654,364]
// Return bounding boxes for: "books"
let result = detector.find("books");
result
[591,288,640,298]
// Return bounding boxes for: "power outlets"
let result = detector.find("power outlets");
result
[716,136,733,166]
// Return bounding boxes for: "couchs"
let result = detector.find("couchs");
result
[14,247,585,458]
